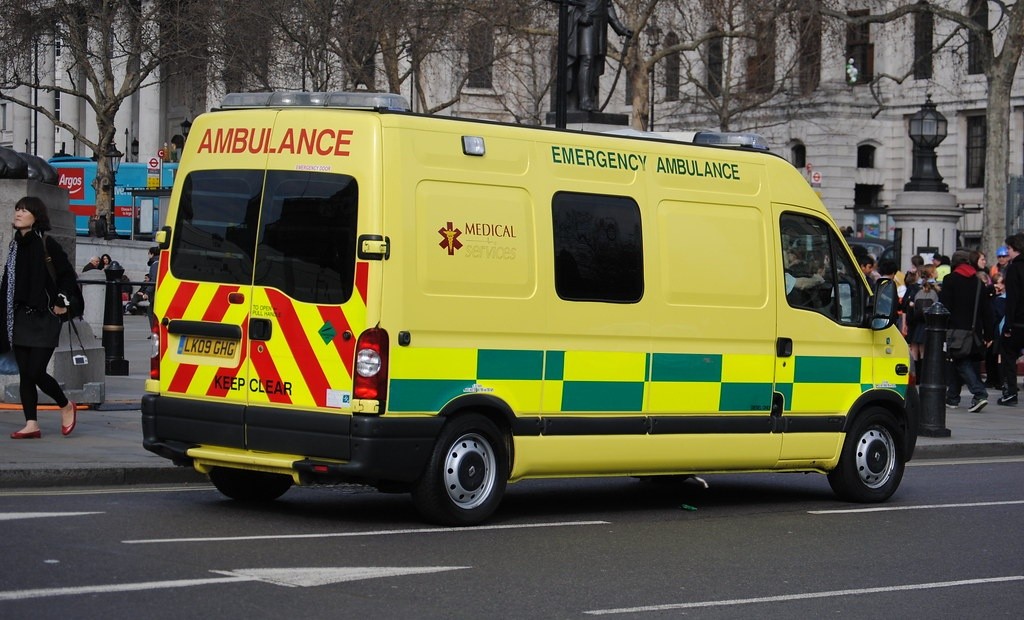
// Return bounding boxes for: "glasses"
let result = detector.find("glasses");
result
[997,255,1007,259]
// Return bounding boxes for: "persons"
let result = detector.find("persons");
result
[82,247,161,340]
[852,232,1024,412]
[0,196,81,439]
[785,273,825,296]
[786,247,801,269]
[840,220,861,240]
[868,224,880,236]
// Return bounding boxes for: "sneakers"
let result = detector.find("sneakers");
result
[968,398,988,413]
[946,398,959,409]
[997,394,1018,405]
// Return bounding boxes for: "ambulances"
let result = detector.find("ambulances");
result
[137,93,914,513]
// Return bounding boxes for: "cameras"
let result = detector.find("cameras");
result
[73,355,88,365]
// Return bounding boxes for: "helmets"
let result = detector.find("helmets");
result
[996,246,1010,256]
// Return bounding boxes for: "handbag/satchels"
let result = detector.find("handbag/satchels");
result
[945,329,974,360]
[43,233,85,322]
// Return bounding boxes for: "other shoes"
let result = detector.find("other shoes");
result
[147,335,151,340]
[1001,383,1019,395]
[986,382,993,388]
[125,311,131,315]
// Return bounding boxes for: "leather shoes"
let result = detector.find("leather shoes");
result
[11,428,41,439]
[62,400,77,436]
